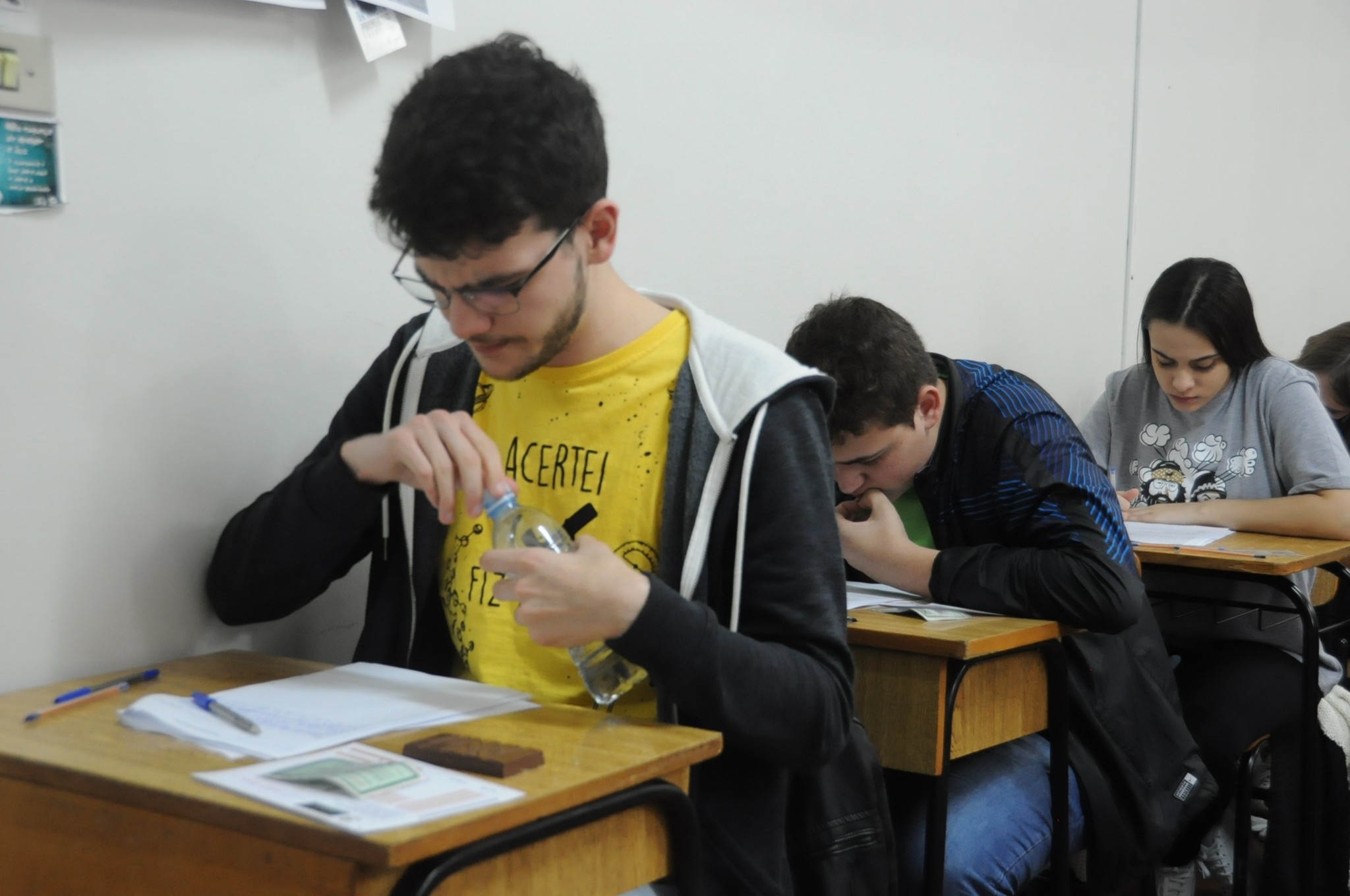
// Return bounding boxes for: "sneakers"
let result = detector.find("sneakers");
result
[1153,859,1194,896]
[1195,821,1234,895]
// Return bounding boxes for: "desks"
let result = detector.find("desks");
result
[844,596,1112,896]
[1125,522,1349,896]
[0,646,725,896]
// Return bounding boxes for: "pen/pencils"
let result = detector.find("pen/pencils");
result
[1131,542,1266,558]
[55,668,160,704]
[25,682,130,721]
[193,692,260,735]
[1110,468,1116,489]
[846,616,856,622]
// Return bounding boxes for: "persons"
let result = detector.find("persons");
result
[786,298,1219,896]
[1080,258,1350,896]
[206,31,854,896]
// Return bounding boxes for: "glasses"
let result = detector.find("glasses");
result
[392,209,580,316]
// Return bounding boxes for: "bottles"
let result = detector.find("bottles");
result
[477,486,651,705]
